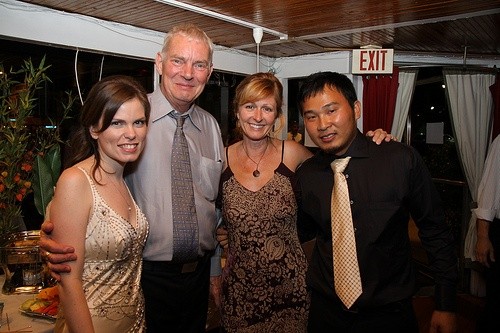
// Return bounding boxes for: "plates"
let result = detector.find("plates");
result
[17,294,58,319]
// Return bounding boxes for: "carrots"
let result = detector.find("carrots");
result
[33,301,59,315]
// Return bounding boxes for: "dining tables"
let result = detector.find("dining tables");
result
[0,274,56,333]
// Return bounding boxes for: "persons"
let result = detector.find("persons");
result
[220,72,398,333]
[292,71,458,333]
[50,75,151,333]
[40,22,224,333]
[464,134,500,333]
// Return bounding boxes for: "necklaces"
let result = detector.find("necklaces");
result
[104,171,132,211]
[241,138,270,177]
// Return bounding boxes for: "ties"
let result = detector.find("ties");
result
[168,112,200,254]
[331,156,363,310]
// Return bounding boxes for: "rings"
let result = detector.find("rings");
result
[43,250,51,261]
